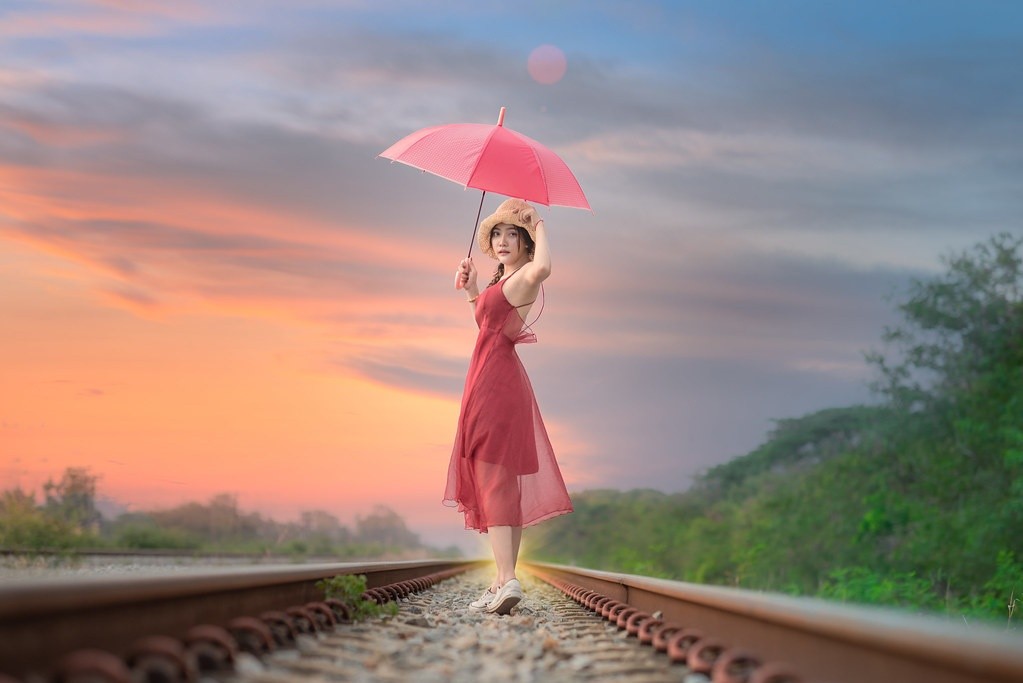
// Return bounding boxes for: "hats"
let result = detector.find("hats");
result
[477,198,537,260]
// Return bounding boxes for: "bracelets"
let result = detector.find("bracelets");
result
[468,296,477,303]
[535,219,543,231]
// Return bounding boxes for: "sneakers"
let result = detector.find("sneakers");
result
[468,586,504,612]
[487,579,522,614]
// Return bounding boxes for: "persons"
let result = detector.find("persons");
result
[441,198,574,615]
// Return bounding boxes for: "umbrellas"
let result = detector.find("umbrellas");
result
[375,108,594,292]
